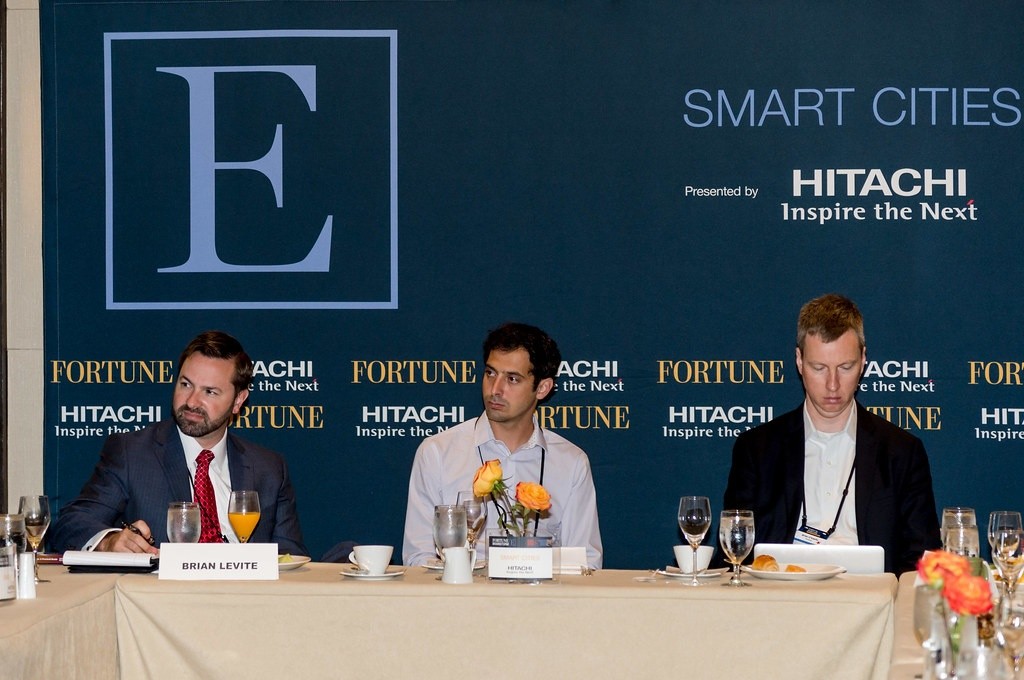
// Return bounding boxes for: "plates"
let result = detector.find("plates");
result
[652,567,731,578]
[278,554,312,571]
[421,561,487,569]
[341,569,404,581]
[739,562,847,581]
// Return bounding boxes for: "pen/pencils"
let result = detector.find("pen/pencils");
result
[119,520,155,545]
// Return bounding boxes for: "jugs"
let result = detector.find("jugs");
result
[439,547,476,585]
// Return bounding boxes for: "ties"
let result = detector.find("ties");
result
[190,450,223,543]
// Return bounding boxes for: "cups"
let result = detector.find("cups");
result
[348,545,394,575]
[0,513,29,575]
[166,502,202,544]
[672,545,714,573]
[432,504,467,551]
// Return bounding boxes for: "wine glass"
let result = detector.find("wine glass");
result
[15,493,53,583]
[455,490,488,549]
[718,509,755,587]
[905,509,1024,679]
[678,496,712,587]
[227,490,261,543]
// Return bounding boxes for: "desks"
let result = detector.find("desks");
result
[0,563,926,680]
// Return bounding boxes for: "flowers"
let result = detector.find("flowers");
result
[916,550,995,678]
[473,459,553,539]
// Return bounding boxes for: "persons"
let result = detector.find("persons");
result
[51,333,359,563]
[709,292,944,577]
[402,320,603,569]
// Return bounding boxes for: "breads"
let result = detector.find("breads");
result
[750,554,780,572]
[784,565,807,572]
